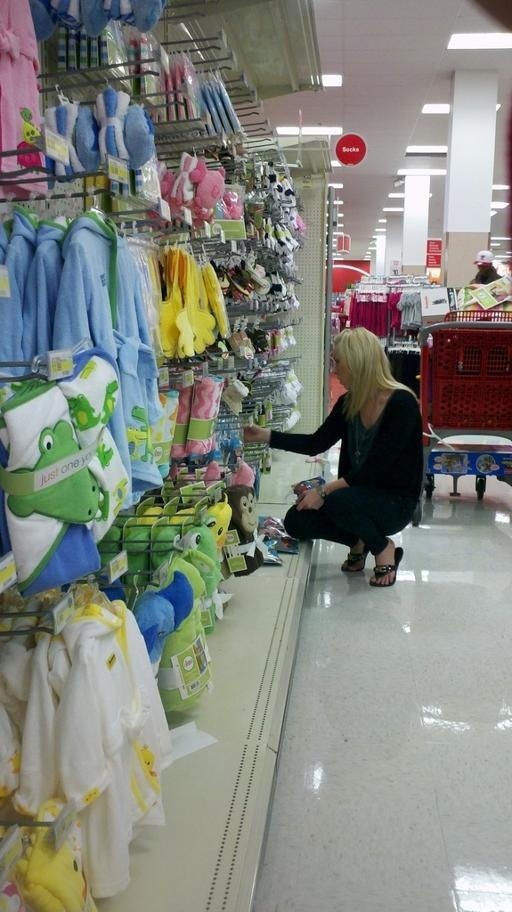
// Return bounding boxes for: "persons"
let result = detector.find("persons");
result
[242,326,425,587]
[470,251,502,284]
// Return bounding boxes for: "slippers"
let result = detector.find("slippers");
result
[369,547,404,587]
[341,541,369,571]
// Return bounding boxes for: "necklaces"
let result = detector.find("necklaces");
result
[354,408,371,464]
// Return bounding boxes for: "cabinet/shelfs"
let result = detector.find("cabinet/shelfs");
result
[3,0,331,910]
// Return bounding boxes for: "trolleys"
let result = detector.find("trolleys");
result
[412,310,512,527]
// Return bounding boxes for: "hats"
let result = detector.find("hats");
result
[474,250,494,266]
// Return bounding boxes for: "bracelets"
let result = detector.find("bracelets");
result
[316,486,327,497]
